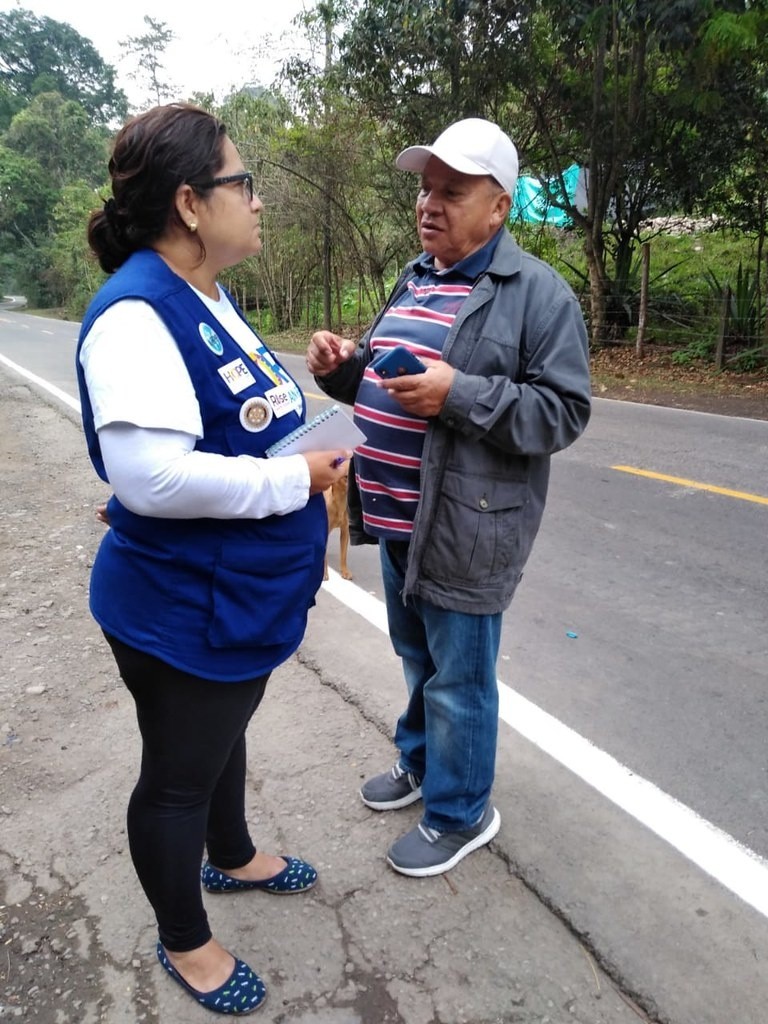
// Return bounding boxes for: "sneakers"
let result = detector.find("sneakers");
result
[359,762,501,878]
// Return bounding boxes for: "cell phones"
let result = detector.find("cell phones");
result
[373,345,426,379]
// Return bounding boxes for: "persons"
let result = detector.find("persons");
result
[303,116,596,877]
[77,102,349,1016]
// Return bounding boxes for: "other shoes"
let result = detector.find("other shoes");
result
[157,855,321,1016]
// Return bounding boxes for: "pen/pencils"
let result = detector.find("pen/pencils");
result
[333,454,346,467]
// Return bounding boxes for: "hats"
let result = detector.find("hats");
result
[396,117,519,197]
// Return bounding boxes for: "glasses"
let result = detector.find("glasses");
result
[188,170,254,201]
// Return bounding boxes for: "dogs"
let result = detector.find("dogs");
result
[321,460,353,580]
